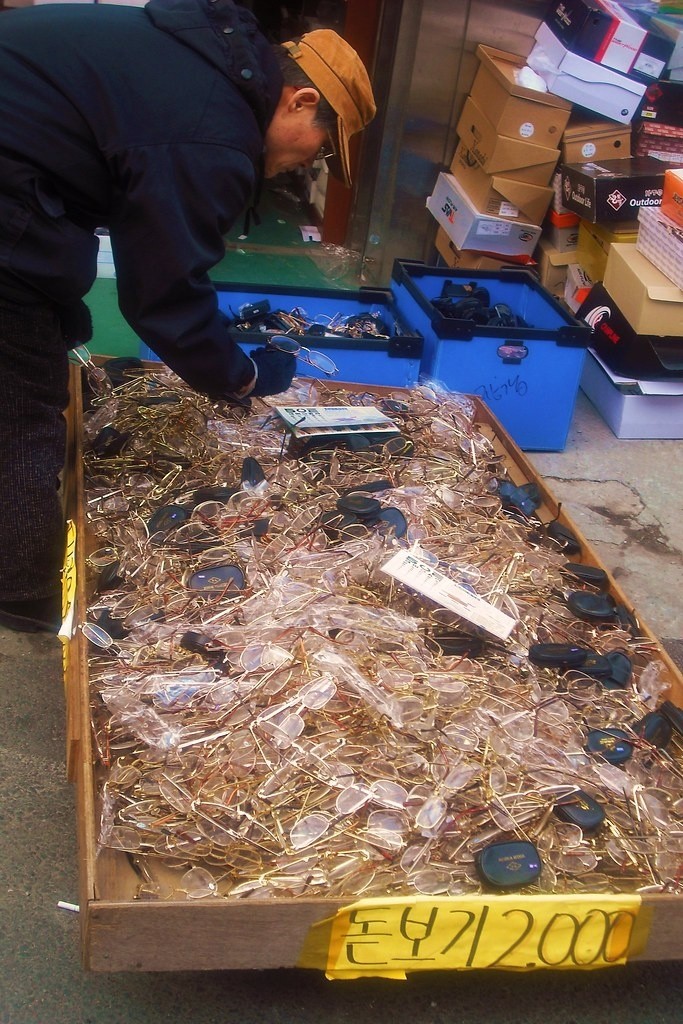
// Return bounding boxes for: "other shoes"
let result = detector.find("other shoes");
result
[0,590,62,634]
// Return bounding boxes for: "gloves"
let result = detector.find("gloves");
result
[249,343,297,398]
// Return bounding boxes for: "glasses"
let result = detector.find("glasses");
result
[267,335,339,375]
[69,338,683,901]
[316,105,336,161]
[222,299,392,339]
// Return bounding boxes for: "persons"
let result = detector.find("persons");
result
[0,0,378,635]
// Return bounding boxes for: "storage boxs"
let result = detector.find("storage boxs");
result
[141,281,422,392]
[392,1,683,450]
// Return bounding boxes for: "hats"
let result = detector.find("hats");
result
[281,29,376,189]
